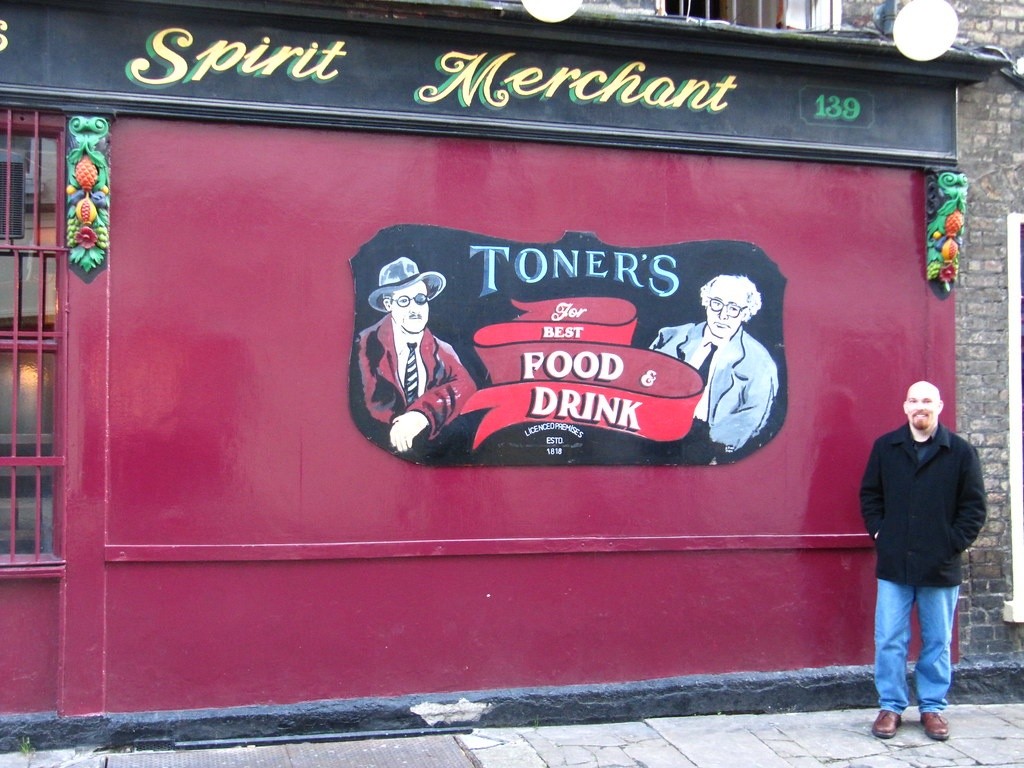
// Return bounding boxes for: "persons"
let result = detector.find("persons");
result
[860,380,988,738]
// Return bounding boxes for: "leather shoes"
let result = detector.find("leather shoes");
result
[920,712,949,740]
[872,709,901,739]
